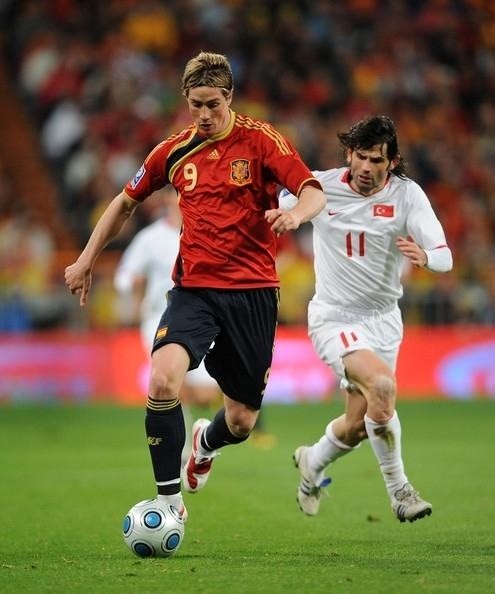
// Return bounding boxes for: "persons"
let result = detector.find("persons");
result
[115,181,223,468]
[62,52,327,520]
[279,114,453,522]
[0,0,495,332]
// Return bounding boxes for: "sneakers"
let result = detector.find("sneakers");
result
[184,418,217,492]
[390,484,431,523]
[177,500,187,523]
[292,445,331,514]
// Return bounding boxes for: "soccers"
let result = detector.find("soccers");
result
[123,499,185,557]
